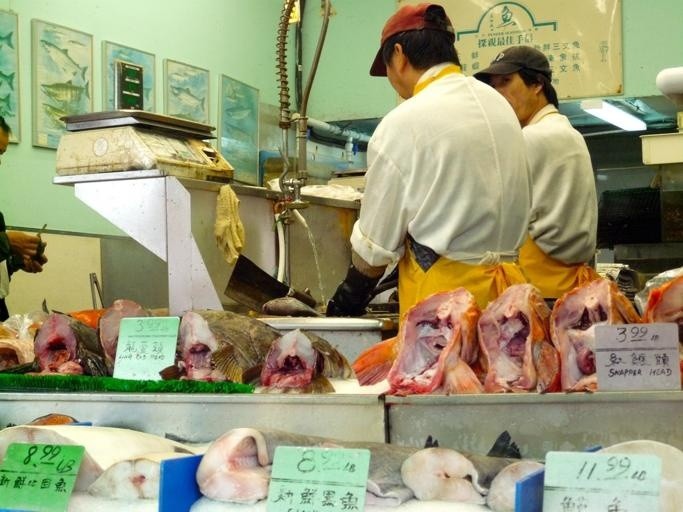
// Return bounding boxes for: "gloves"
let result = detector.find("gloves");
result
[327,263,384,318]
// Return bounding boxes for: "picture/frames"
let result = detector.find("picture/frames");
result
[30,18,94,149]
[163,58,210,127]
[101,40,156,113]
[216,73,261,187]
[0,10,22,145]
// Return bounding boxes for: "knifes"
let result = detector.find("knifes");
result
[224,254,316,314]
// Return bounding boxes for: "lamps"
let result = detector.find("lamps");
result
[583,99,647,131]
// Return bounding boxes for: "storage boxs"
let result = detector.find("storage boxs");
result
[639,132,683,165]
[1,378,385,444]
[383,387,683,459]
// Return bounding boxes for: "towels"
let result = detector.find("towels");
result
[215,185,245,264]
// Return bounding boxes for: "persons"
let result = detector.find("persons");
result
[325,2,534,354]
[471,46,598,308]
[1,115,48,324]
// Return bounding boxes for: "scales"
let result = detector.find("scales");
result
[52,107,235,182]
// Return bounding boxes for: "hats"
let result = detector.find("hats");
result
[370,3,456,76]
[474,46,552,83]
